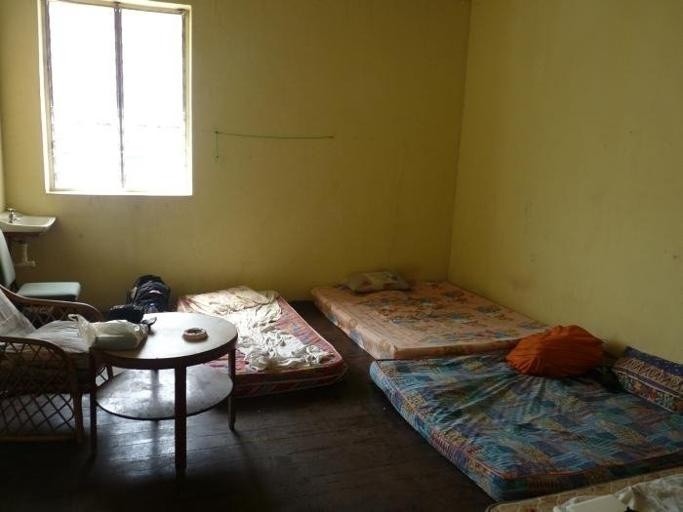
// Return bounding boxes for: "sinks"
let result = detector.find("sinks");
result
[0,214,56,232]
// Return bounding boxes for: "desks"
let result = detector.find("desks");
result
[90,312,238,470]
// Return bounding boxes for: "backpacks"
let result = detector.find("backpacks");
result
[127,274,172,314]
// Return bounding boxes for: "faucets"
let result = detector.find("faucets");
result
[8,207,20,223]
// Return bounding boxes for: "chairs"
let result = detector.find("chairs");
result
[0,283,113,449]
[0,227,81,301]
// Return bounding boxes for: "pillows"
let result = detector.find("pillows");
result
[185,286,270,314]
[344,271,410,293]
[608,346,683,414]
